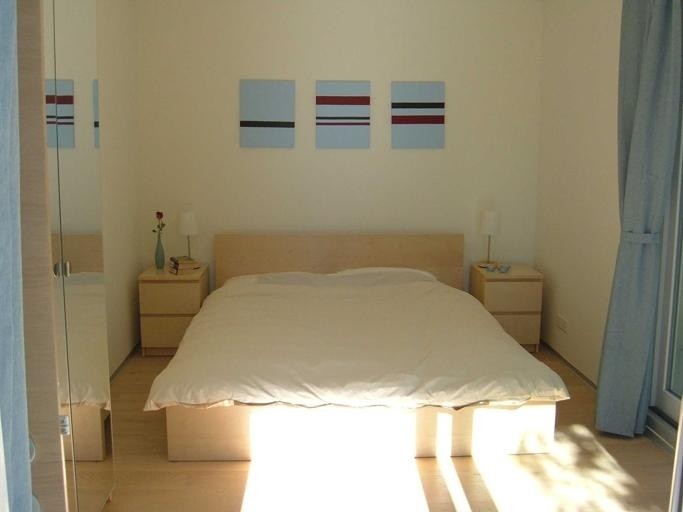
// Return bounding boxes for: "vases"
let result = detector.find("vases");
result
[156,235,164,269]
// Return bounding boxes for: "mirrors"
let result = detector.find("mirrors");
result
[40,0,117,416]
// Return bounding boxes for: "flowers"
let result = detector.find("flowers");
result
[151,210,166,235]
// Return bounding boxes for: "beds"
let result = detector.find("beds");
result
[146,229,570,460]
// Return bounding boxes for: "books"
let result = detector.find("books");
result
[167,255,197,276]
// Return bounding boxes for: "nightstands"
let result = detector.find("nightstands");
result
[135,263,209,358]
[469,258,543,356]
